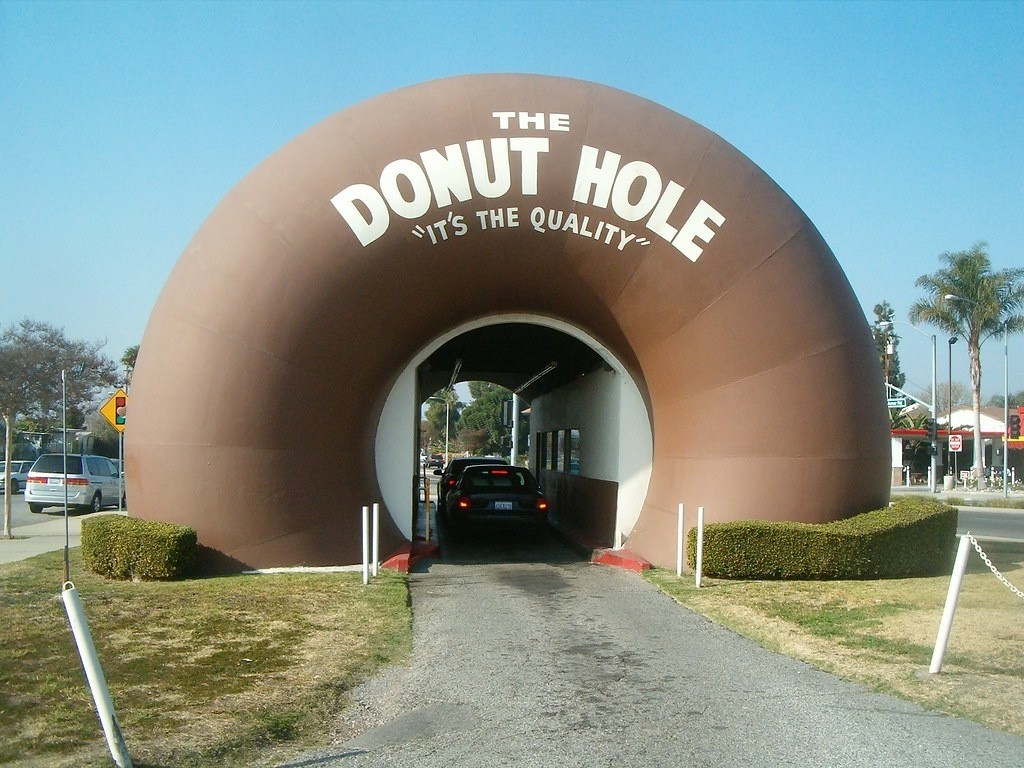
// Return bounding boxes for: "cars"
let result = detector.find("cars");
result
[433,457,510,518]
[425,454,444,468]
[444,463,549,545]
[106,459,124,477]
[0,461,37,494]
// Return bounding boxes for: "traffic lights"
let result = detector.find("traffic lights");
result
[116,396,127,426]
[922,417,937,441]
[1008,414,1022,440]
[926,446,937,457]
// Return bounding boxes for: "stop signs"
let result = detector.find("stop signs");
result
[949,435,962,452]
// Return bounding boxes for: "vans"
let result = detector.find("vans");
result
[25,452,128,514]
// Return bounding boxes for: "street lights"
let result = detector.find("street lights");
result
[429,396,449,468]
[880,320,937,494]
[942,336,958,489]
[945,292,1009,499]
